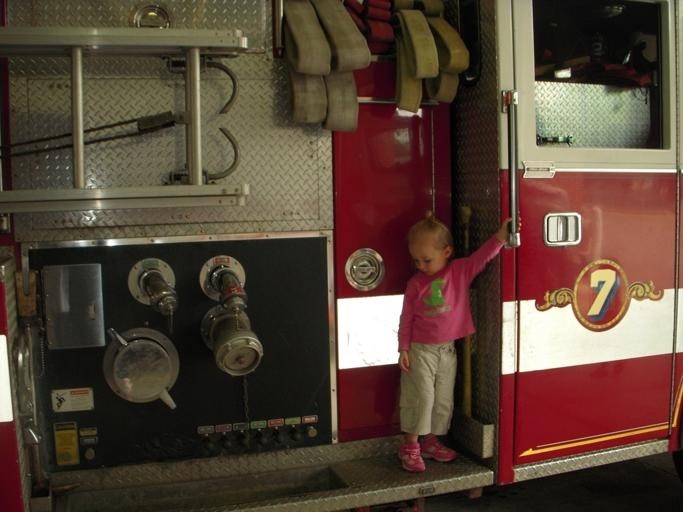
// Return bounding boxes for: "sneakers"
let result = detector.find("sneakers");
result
[420,438,457,461]
[399,442,426,472]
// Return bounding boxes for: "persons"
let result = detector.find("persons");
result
[394,213,523,473]
